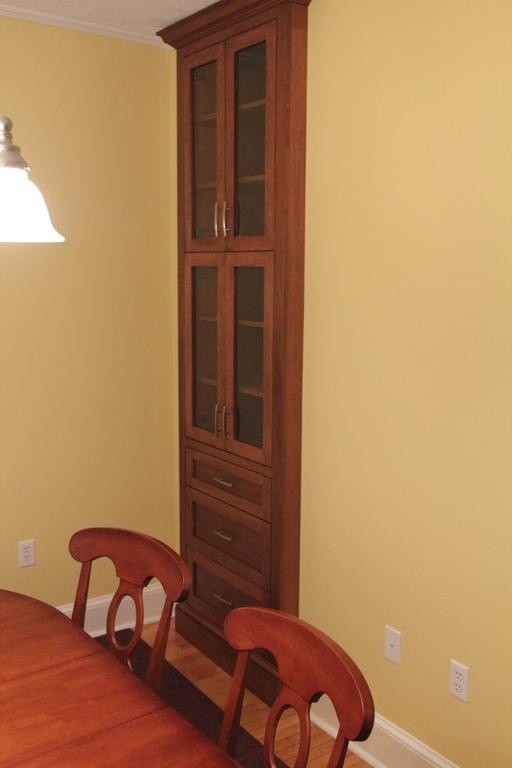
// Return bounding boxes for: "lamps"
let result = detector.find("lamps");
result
[0,116,65,243]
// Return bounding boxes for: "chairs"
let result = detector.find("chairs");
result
[0,590,242,768]
[217,607,374,768]
[68,527,192,699]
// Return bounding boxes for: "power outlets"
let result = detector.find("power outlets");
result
[18,540,35,567]
[449,659,469,703]
